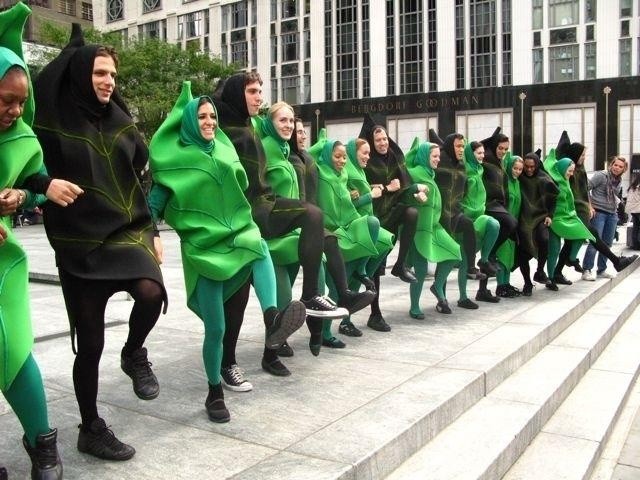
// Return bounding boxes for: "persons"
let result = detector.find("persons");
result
[495,151,522,298]
[457,137,499,310]
[287,117,375,357]
[0,45,64,479]
[475,127,519,303]
[553,144,640,284]
[511,149,552,296]
[365,125,428,332]
[339,138,395,336]
[401,138,462,320]
[216,73,349,393]
[624,174,640,251]
[316,141,380,348]
[583,156,628,282]
[260,102,326,376]
[30,43,168,462]
[149,96,306,425]
[544,149,597,290]
[435,133,486,314]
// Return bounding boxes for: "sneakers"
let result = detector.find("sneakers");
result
[221,365,252,392]
[497,288,513,297]
[476,291,500,303]
[522,284,532,296]
[598,272,615,278]
[555,277,570,285]
[567,259,583,272]
[505,285,518,295]
[546,281,557,290]
[534,273,550,284]
[467,271,486,280]
[205,384,231,423]
[121,346,160,399]
[22,428,63,480]
[266,301,305,350]
[581,270,594,281]
[77,420,135,460]
[615,255,638,272]
[458,299,479,309]
[299,296,348,319]
[339,290,374,315]
[481,262,497,276]
[436,303,451,314]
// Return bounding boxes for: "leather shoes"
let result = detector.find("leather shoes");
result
[430,285,446,304]
[391,264,417,284]
[322,337,346,348]
[277,340,293,356]
[261,357,290,377]
[306,315,322,356]
[410,309,424,319]
[367,315,390,331]
[339,323,363,336]
